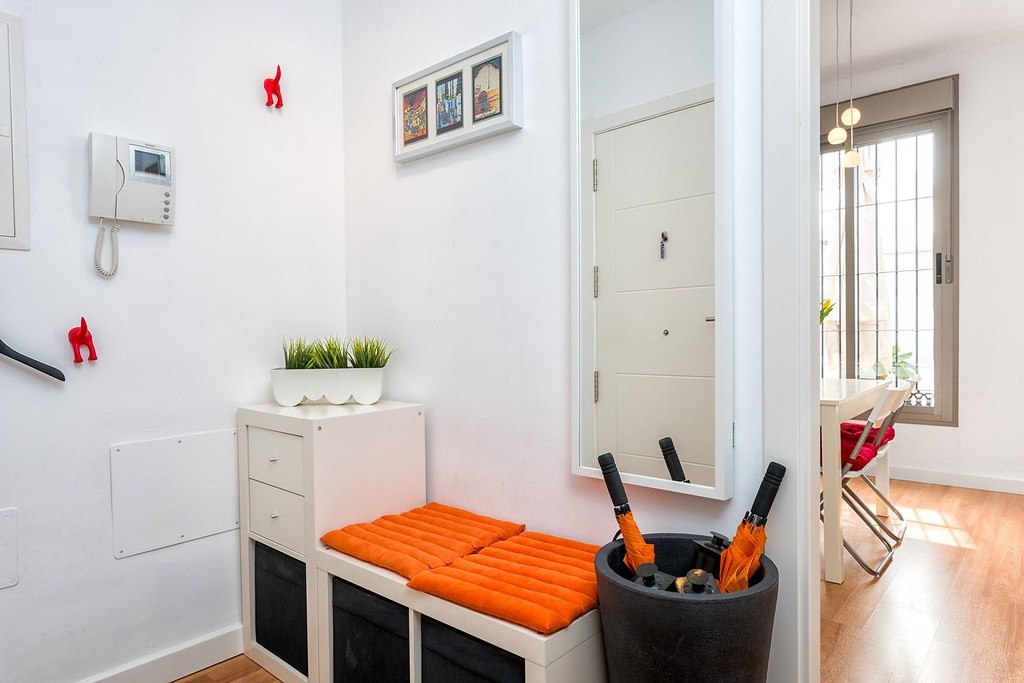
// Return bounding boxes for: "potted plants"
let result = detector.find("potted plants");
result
[267,339,390,406]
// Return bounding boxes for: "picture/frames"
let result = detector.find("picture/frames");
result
[391,29,521,165]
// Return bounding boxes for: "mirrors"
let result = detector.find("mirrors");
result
[569,1,736,502]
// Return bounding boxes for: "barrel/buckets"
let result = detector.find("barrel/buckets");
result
[593,532,779,683]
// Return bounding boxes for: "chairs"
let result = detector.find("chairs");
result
[820,375,919,576]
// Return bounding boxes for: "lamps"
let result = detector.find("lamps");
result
[828,0,861,168]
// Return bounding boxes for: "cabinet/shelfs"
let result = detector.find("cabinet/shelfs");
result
[320,504,605,683]
[238,403,424,683]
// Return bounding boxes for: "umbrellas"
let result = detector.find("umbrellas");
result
[598,454,655,570]
[719,462,786,592]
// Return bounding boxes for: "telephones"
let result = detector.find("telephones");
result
[88,132,176,226]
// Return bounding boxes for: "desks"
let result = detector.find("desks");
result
[821,379,894,585]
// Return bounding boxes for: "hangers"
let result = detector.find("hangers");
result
[0,334,65,382]
